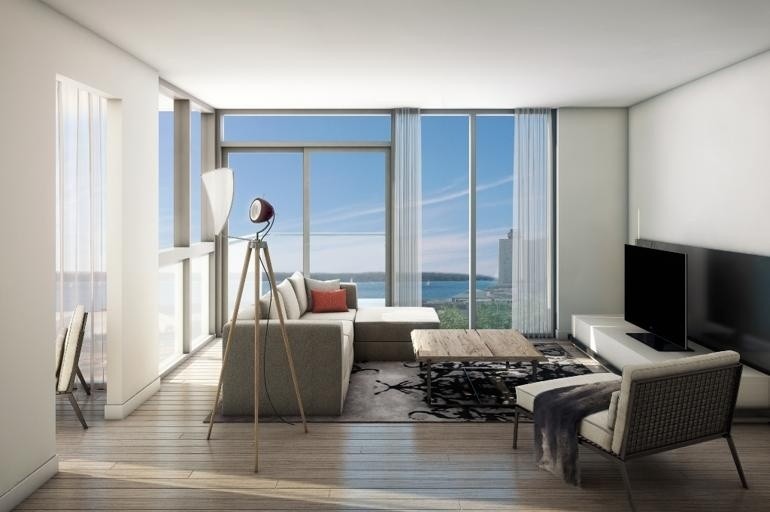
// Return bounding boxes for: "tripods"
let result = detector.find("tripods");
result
[207,240,309,474]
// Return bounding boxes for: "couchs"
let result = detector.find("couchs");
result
[221,283,442,416]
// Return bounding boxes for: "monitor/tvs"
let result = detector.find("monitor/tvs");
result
[625,244,695,351]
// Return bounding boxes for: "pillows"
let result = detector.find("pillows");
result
[260,271,349,320]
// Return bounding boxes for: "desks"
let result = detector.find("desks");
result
[567,313,769,417]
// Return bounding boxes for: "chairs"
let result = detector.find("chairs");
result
[513,350,750,510]
[56,304,90,431]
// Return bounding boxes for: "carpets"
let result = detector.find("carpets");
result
[202,339,609,425]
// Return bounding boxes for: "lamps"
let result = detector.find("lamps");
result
[200,168,308,472]
